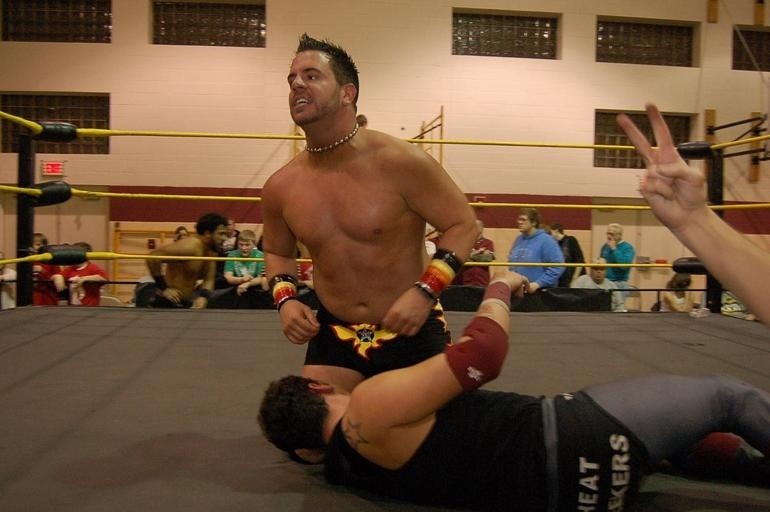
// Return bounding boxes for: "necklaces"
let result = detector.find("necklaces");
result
[303,123,358,154]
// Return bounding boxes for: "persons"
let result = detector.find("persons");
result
[0,251,18,311]
[250,267,770,510]
[15,232,112,308]
[615,102,768,327]
[415,206,637,313]
[659,272,696,314]
[128,206,316,310]
[260,32,483,466]
